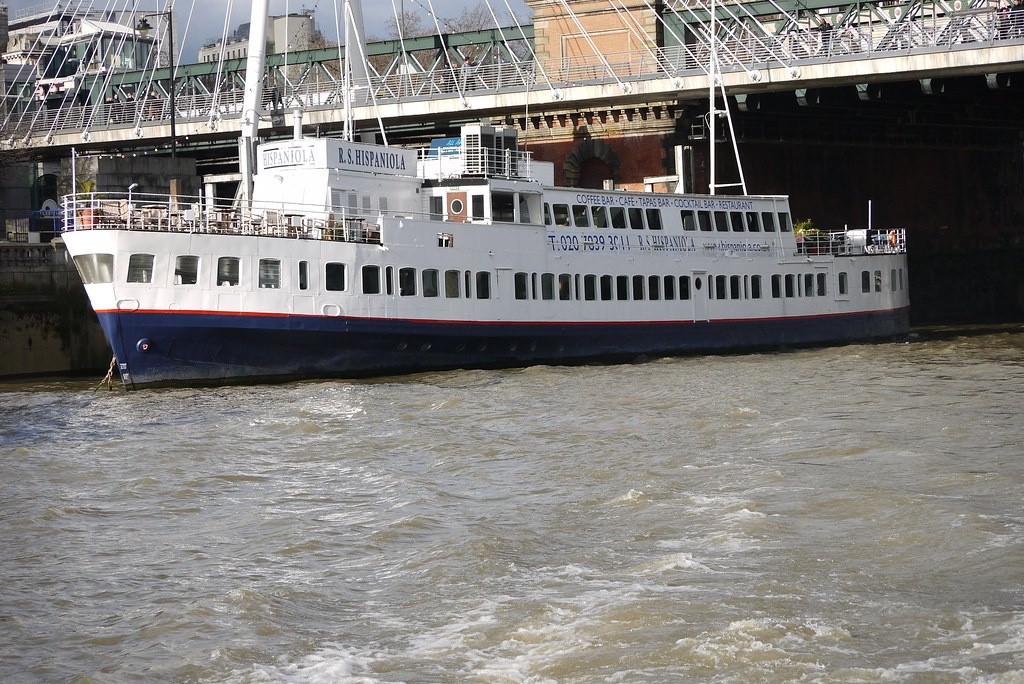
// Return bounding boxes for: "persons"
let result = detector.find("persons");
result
[817,18,833,56]
[104,89,165,124]
[440,56,479,93]
[1010,0,1024,39]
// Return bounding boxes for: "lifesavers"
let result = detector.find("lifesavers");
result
[888,231,896,245]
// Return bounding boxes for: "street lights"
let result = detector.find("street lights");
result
[134,4,182,226]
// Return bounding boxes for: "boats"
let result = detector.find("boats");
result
[55,1,910,386]
